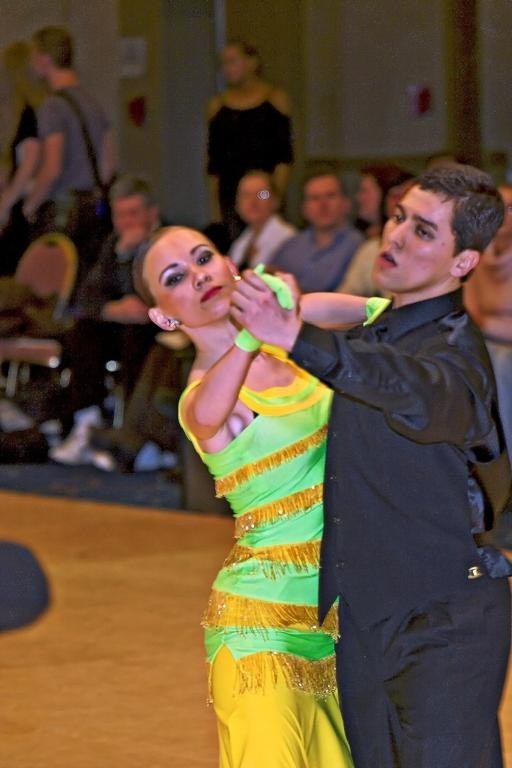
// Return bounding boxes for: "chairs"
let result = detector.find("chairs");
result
[1,232,83,384]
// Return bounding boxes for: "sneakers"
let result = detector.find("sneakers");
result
[48,404,104,466]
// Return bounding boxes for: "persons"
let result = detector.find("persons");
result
[229,159,512,768]
[134,220,399,768]
